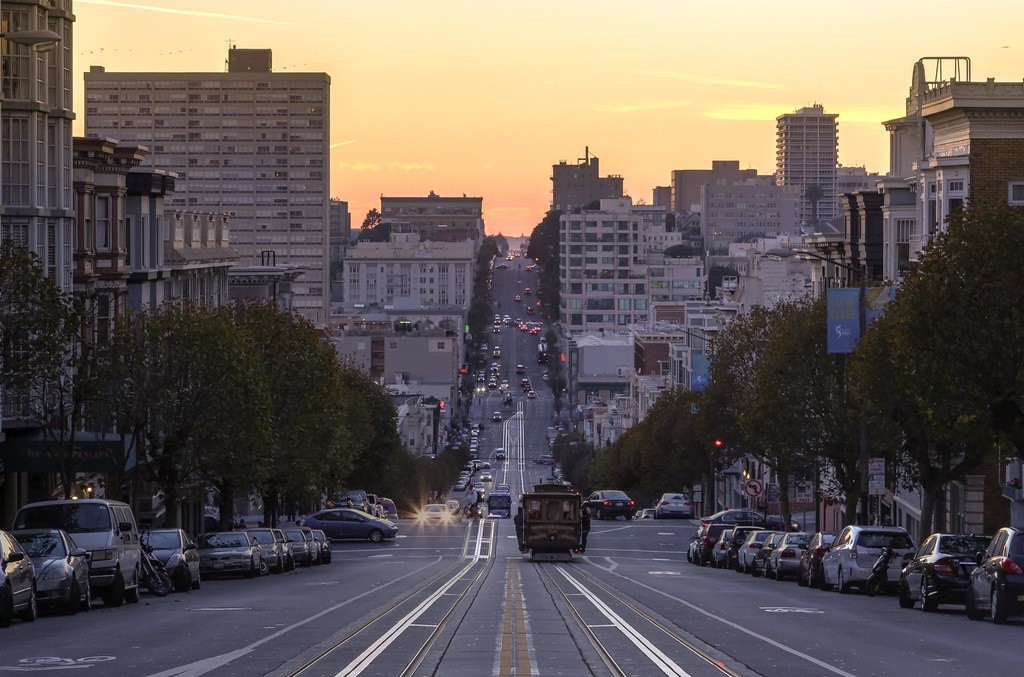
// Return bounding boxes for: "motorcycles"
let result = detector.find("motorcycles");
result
[139,528,173,597]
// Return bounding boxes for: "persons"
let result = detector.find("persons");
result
[258,521,264,527]
[286,499,296,522]
[377,502,383,517]
[347,497,353,508]
[326,500,334,508]
[237,519,247,528]
[514,507,523,548]
[362,499,369,511]
[470,485,479,522]
[582,507,590,551]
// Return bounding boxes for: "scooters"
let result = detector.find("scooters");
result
[863,547,915,597]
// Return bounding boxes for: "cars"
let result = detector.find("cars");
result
[477,247,550,406]
[332,488,399,521]
[687,522,840,589]
[301,508,398,543]
[137,529,201,593]
[447,500,460,516]
[231,527,333,574]
[424,504,448,522]
[582,490,635,521]
[965,527,1024,623]
[534,426,571,486]
[493,411,502,421]
[194,531,263,580]
[496,484,510,491]
[452,423,492,501]
[654,492,694,520]
[699,507,800,533]
[8,529,91,616]
[466,506,483,519]
[633,509,655,520]
[900,533,995,611]
[0,529,38,627]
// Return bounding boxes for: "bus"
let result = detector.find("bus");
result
[515,483,589,556]
[487,492,512,520]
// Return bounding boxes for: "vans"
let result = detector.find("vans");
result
[496,449,506,460]
[10,498,142,607]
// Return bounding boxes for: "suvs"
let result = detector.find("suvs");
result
[820,524,920,594]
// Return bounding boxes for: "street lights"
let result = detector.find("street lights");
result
[657,323,715,516]
[763,248,869,526]
